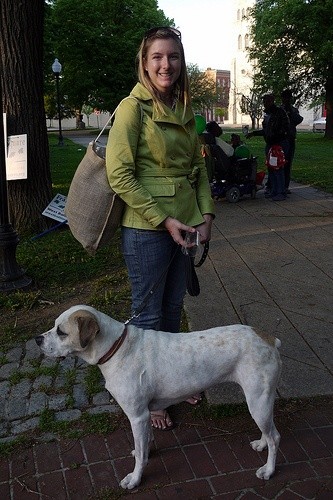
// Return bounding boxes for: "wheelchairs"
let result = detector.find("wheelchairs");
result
[199,133,259,203]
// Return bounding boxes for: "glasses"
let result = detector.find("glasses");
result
[145,26,181,42]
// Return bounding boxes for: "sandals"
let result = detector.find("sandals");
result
[150,410,173,432]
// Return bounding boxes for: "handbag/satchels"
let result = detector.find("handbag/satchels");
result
[65,96,143,256]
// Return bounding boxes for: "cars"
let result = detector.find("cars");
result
[312,117,326,130]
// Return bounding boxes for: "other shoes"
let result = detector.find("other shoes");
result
[272,193,287,201]
[266,194,275,201]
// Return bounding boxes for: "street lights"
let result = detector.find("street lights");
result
[52,58,65,145]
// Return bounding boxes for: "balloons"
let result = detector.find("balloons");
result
[236,144,250,157]
[194,114,206,135]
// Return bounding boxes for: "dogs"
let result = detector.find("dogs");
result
[36,305,287,490]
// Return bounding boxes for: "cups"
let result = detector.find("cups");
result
[181,230,202,257]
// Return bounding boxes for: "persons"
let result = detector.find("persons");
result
[280,91,303,194]
[106,26,216,431]
[244,94,291,197]
[205,121,259,187]
[265,135,286,201]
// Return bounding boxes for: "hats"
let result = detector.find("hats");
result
[261,95,274,101]
